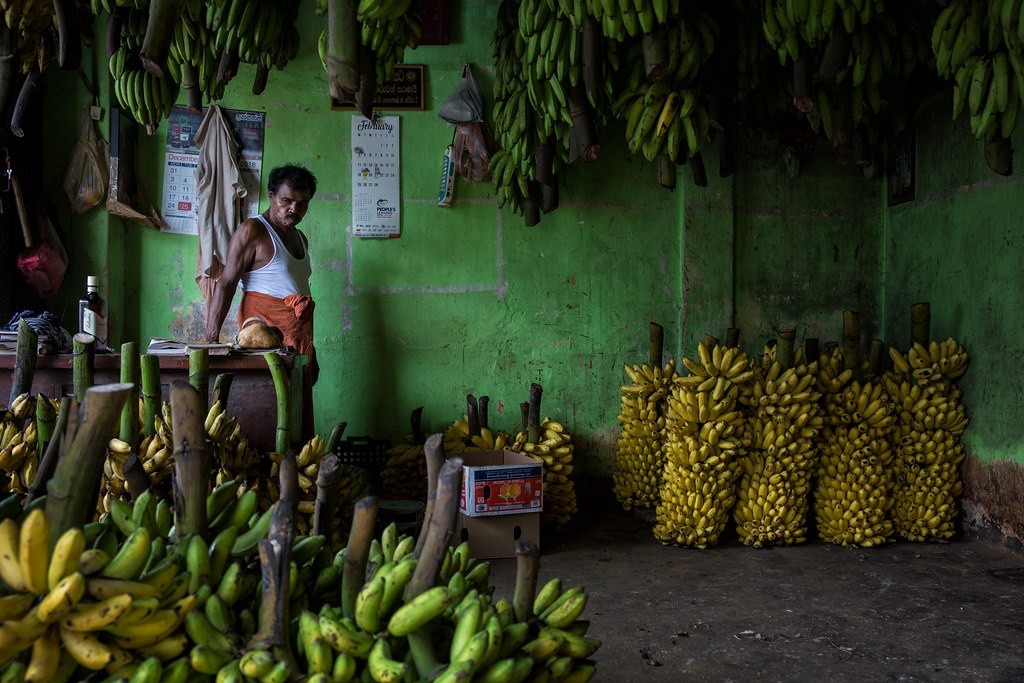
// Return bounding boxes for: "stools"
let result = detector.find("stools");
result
[377,498,424,534]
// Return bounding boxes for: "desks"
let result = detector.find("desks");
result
[0,349,309,444]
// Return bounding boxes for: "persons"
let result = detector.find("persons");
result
[206,163,319,446]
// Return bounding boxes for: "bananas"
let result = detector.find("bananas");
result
[0,0,424,133]
[486,1,1024,227]
[0,315,601,683]
[611,303,971,550]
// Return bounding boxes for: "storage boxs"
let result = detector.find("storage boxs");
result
[453,513,540,559]
[334,435,392,475]
[446,448,542,517]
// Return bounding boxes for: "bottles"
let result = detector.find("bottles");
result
[78,275,108,352]
